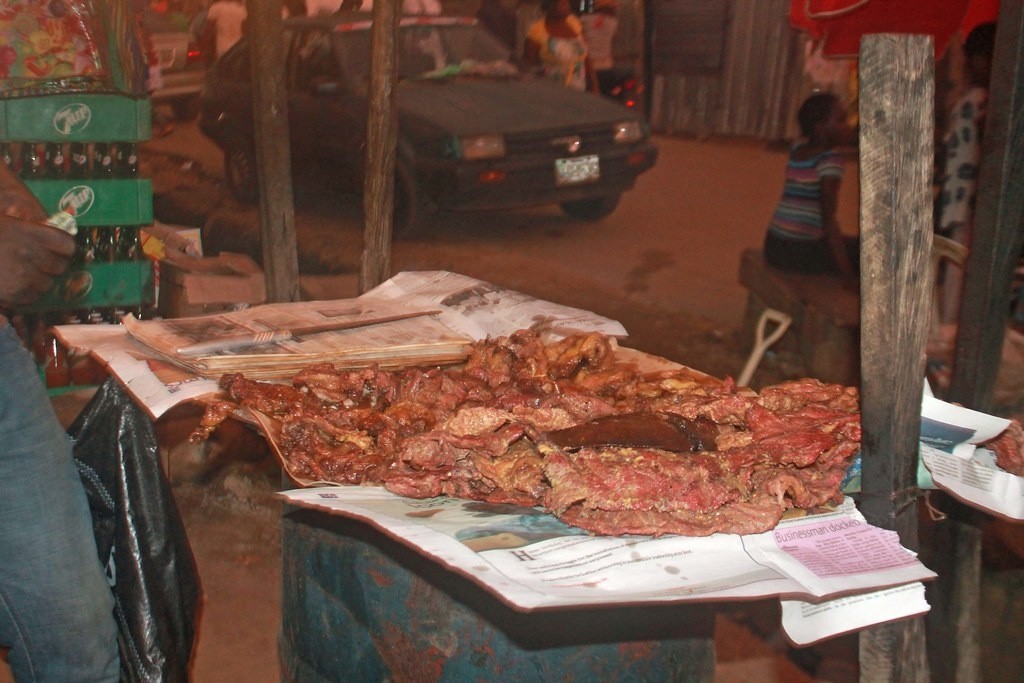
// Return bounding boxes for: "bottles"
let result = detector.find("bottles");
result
[2,139,162,388]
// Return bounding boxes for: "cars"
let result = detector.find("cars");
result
[199,11,653,246]
[134,7,207,118]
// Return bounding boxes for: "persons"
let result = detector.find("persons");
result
[1,162,128,683]
[939,19,1024,341]
[765,89,861,280]
[134,0,627,126]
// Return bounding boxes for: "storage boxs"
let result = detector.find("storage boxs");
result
[258,456,710,681]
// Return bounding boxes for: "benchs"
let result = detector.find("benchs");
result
[731,252,859,383]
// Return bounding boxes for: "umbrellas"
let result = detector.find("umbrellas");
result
[782,0,1022,62]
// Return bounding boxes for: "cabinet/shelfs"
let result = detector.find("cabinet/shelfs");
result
[1,75,158,397]
